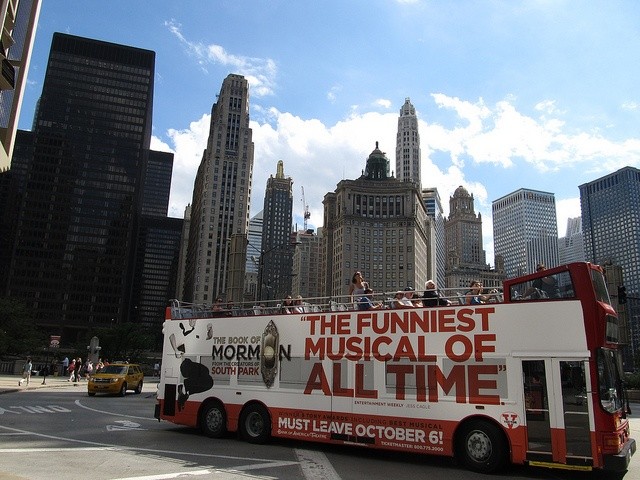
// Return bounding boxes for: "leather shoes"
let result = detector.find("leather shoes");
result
[178,385,189,412]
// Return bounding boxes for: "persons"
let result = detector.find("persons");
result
[464,280,480,305]
[61,357,110,382]
[280,295,292,315]
[521,264,561,299]
[391,286,405,307]
[477,283,496,303]
[349,271,370,310]
[50,355,57,372]
[212,298,224,317]
[124,357,131,364]
[152,361,160,378]
[401,284,416,307]
[225,301,235,315]
[412,290,423,308]
[360,288,382,310]
[19,357,32,387]
[422,282,452,306]
[293,293,305,315]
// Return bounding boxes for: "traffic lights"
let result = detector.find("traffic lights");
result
[617,285,628,304]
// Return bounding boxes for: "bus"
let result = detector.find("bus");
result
[153,261,638,479]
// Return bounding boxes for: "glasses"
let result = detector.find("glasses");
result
[218,300,222,303]
[536,267,544,271]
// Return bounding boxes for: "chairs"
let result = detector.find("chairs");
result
[188,298,396,319]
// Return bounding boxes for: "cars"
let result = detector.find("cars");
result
[87,363,144,398]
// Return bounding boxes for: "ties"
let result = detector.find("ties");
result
[180,323,194,336]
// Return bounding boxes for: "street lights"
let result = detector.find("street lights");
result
[261,273,299,306]
[41,347,49,385]
[244,239,303,306]
